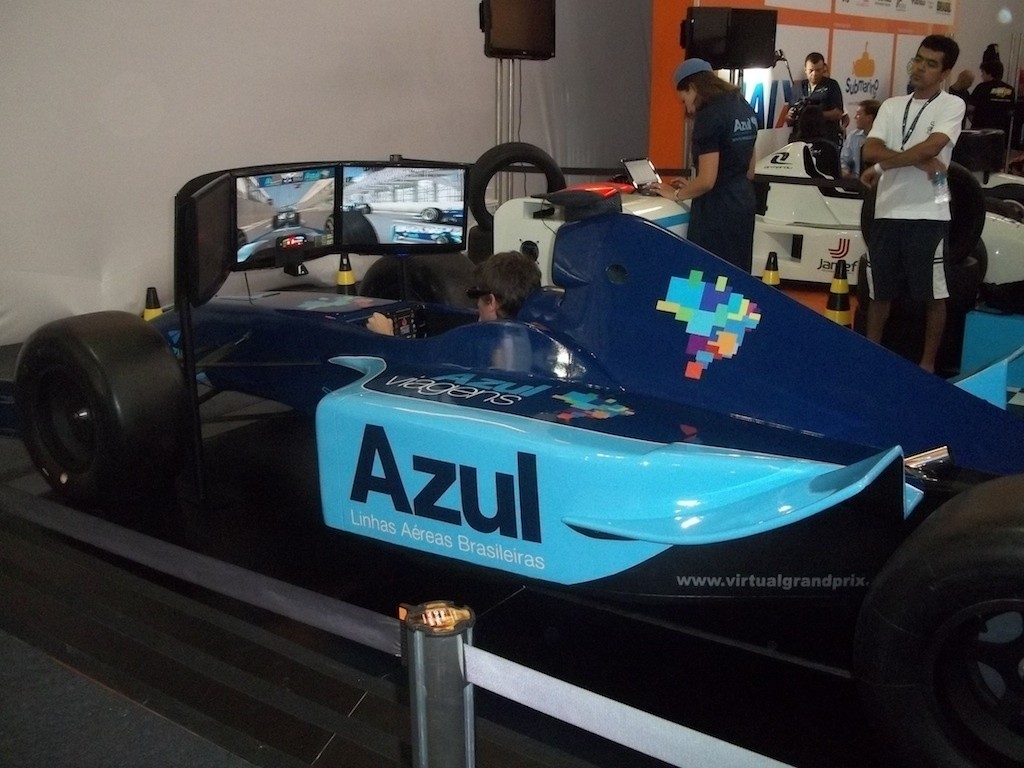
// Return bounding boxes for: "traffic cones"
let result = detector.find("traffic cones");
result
[824,260,852,330]
[760,251,781,292]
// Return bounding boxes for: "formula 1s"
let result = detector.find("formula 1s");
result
[749,138,1024,317]
[15,140,1024,768]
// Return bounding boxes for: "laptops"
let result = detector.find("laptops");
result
[620,157,664,196]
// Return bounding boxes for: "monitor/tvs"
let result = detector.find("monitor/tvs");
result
[179,173,230,309]
[229,162,338,274]
[339,160,470,255]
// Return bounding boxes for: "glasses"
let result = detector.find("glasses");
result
[465,286,490,299]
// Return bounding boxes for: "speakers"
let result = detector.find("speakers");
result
[680,6,778,70]
[478,0,556,61]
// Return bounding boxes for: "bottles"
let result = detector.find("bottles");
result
[933,157,952,203]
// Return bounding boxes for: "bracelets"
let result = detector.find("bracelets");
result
[674,188,682,205]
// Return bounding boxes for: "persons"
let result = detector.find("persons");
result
[647,58,760,275]
[861,34,967,374]
[364,252,543,338]
[785,53,879,179]
[950,69,975,131]
[969,61,1017,177]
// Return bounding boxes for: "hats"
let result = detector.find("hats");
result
[673,58,712,90]
